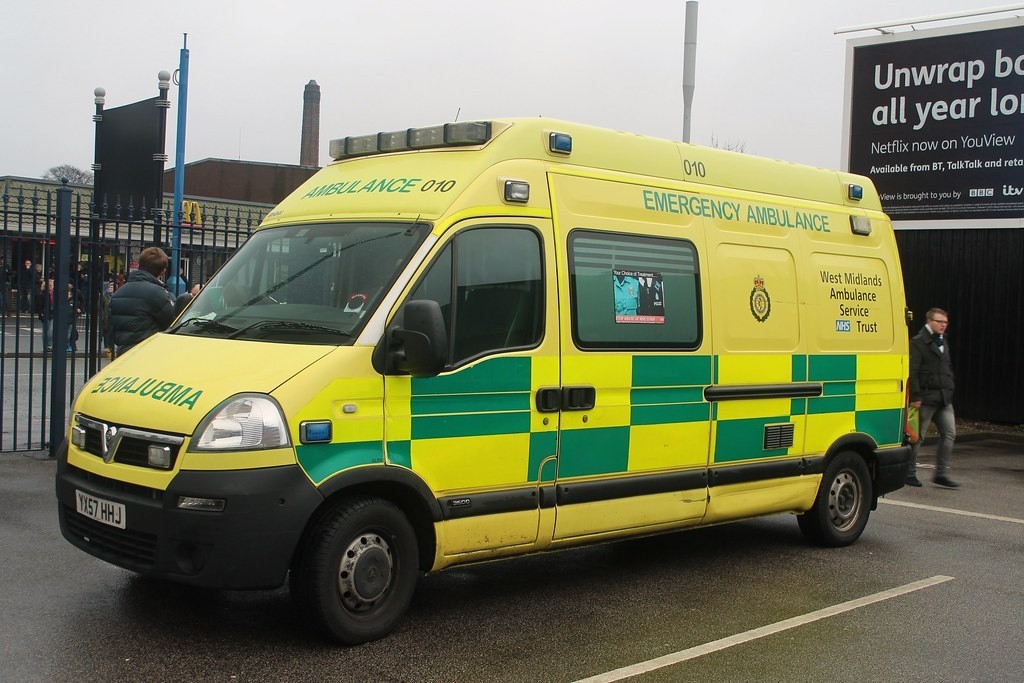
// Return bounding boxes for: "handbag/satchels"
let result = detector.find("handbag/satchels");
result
[903,408,919,443]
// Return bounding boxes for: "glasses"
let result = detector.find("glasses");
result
[931,319,949,325]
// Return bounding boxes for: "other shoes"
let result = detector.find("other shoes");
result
[933,476,959,487]
[905,476,922,487]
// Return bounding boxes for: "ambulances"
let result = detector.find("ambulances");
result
[53,117,917,647]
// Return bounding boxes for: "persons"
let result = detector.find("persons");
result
[171,265,208,321]
[0,255,125,353]
[112,246,177,358]
[909,308,962,488]
[100,282,114,353]
